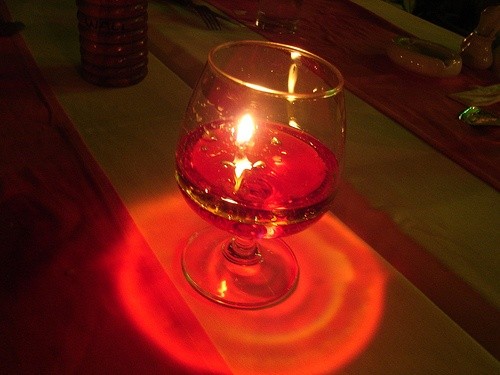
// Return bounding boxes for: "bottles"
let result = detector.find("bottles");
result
[73,0,150,88]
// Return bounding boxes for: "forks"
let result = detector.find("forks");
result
[161,0,222,31]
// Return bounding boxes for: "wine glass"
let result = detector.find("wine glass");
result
[173,40,346,308]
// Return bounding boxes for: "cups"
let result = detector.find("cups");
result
[255,0,302,35]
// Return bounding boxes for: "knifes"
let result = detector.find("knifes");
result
[175,0,245,27]
